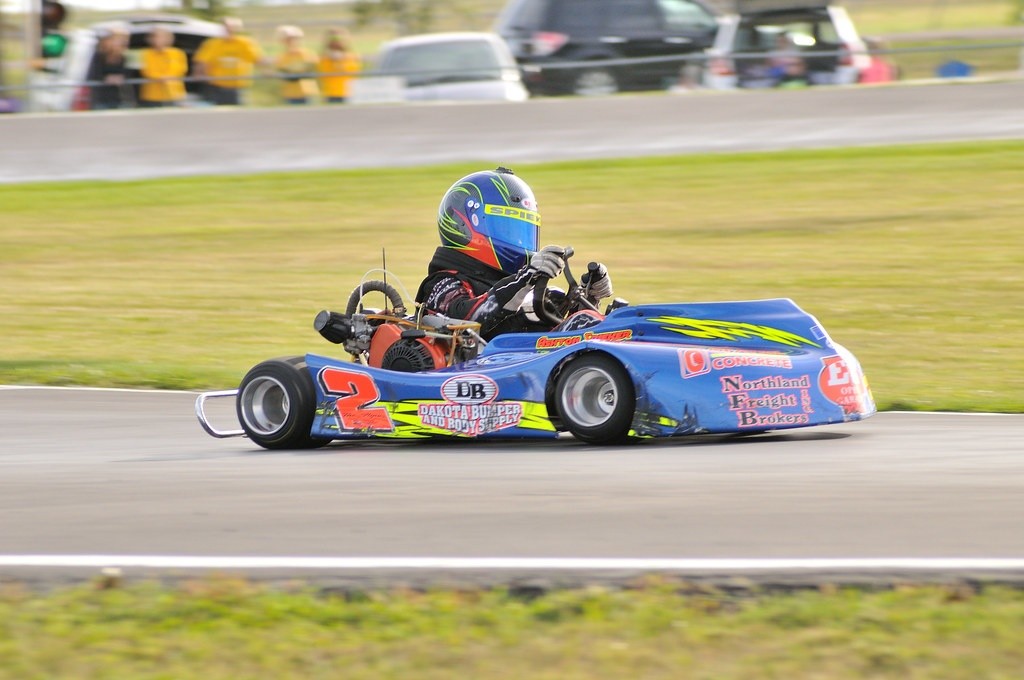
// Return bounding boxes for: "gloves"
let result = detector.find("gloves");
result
[528,245,565,278]
[582,264,613,300]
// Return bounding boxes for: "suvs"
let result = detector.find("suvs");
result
[706,0,875,92]
[27,14,242,112]
[495,1,783,103]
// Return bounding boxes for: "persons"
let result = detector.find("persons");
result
[191,15,277,104]
[83,31,130,111]
[316,27,363,104]
[136,26,189,107]
[414,167,613,357]
[272,23,321,103]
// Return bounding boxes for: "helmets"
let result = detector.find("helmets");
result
[437,167,539,275]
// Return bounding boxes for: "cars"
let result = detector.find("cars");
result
[347,31,527,107]
